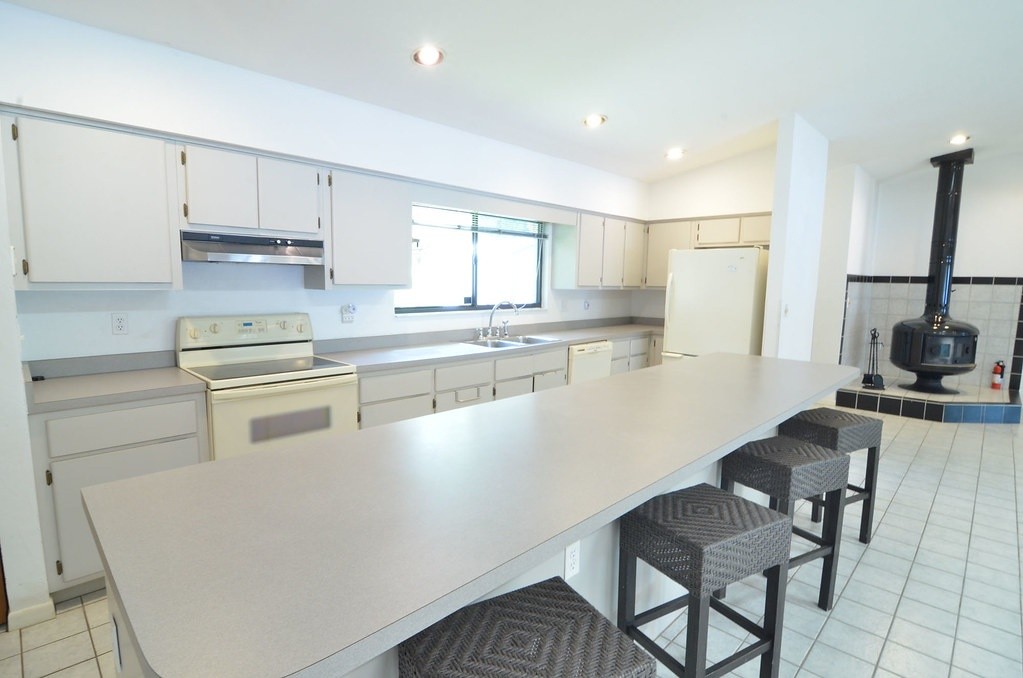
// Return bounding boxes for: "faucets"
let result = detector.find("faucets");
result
[475,301,519,340]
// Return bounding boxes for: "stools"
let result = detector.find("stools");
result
[778,407,883,544]
[398,575,657,678]
[721,436,850,612]
[617,482,793,678]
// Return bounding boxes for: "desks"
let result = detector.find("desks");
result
[79,352,861,678]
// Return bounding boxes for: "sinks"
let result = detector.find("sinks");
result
[502,336,561,345]
[461,340,525,349]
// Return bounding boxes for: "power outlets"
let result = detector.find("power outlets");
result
[112,313,128,335]
[563,542,582,580]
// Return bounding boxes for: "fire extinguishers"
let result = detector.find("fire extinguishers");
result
[992,361,1006,389]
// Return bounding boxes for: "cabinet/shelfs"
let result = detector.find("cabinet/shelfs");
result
[0,100,772,604]
